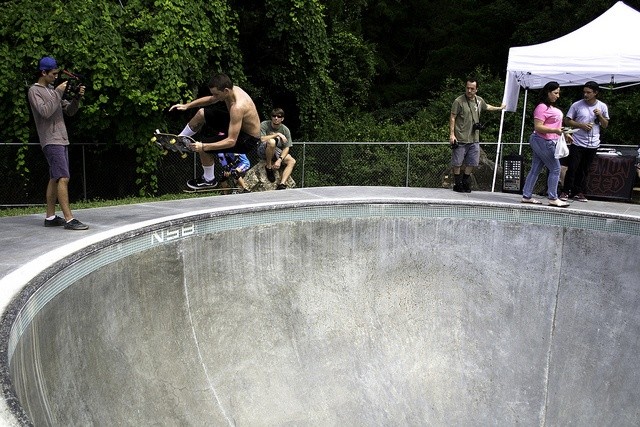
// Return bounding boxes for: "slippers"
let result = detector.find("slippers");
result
[522,197,542,205]
[547,203,569,207]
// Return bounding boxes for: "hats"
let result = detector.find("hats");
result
[39,57,57,70]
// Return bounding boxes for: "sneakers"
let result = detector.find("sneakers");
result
[454,184,464,192]
[277,183,286,189]
[45,215,66,226]
[573,193,587,202]
[187,176,218,190]
[559,191,568,200]
[264,164,275,182]
[64,219,88,229]
[466,185,471,193]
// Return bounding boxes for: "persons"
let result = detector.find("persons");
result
[522,81,571,207]
[155,73,261,189]
[256,108,296,190]
[215,132,251,195]
[28,56,89,230]
[560,81,610,202]
[450,79,506,192]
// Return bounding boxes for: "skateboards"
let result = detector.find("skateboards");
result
[150,133,196,158]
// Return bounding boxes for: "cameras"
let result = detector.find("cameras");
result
[473,123,485,132]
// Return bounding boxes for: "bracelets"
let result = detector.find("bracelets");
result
[279,157,284,161]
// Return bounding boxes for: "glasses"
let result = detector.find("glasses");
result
[272,114,282,117]
